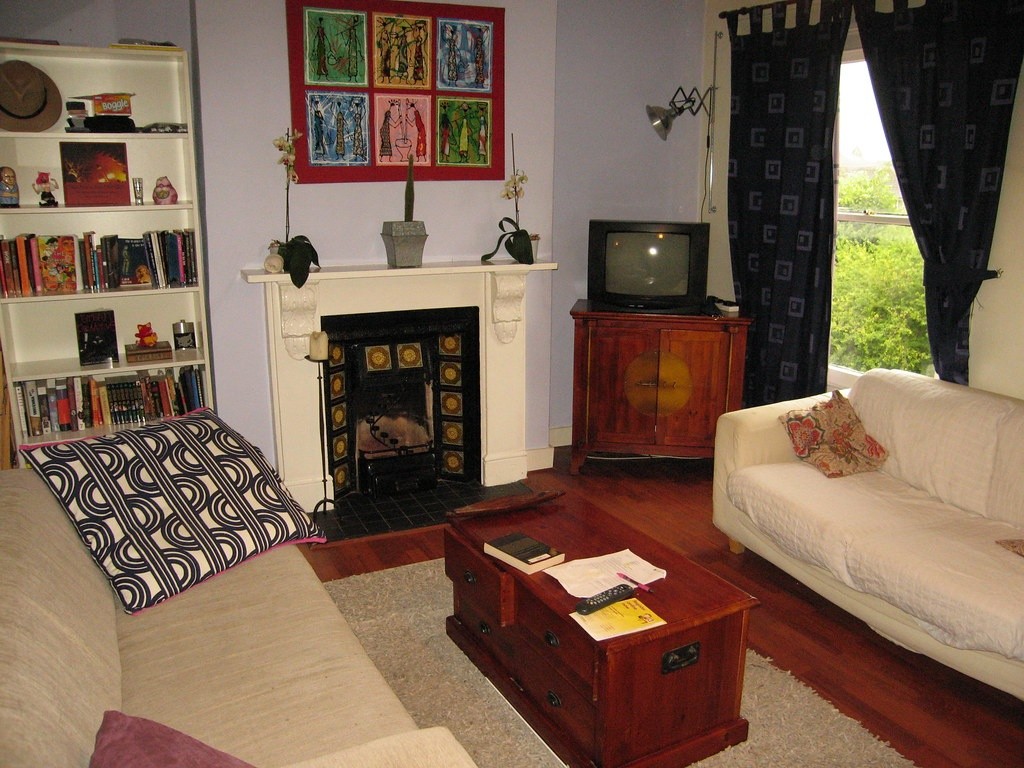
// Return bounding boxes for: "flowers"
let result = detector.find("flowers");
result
[265,125,321,290]
[481,133,534,267]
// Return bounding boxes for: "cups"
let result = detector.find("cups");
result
[132,178,144,205]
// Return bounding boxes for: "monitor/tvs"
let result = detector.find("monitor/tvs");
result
[587,219,710,315]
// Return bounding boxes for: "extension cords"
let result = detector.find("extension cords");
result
[714,302,740,312]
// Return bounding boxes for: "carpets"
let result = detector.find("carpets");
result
[320,551,918,767]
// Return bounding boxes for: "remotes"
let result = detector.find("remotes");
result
[576,584,633,615]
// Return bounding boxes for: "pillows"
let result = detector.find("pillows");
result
[18,405,328,616]
[89,709,252,768]
[996,538,1024,558]
[778,391,887,477]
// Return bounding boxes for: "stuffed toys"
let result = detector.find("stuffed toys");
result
[134,322,158,347]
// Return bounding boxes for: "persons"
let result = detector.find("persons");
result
[0,167,20,208]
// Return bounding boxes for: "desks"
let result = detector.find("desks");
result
[442,484,761,768]
[242,256,558,510]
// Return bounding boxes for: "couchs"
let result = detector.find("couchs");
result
[0,455,478,766]
[711,365,1024,708]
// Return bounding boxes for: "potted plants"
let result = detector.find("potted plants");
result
[379,152,429,268]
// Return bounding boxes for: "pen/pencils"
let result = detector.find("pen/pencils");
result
[616,573,656,594]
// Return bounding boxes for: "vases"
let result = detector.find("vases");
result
[262,243,286,274]
[524,233,541,264]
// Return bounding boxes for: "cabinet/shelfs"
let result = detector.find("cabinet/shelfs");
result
[0,37,217,465]
[567,297,751,477]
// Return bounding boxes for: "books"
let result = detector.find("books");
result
[483,531,565,575]
[125,342,173,362]
[0,228,198,298]
[15,366,206,439]
[73,308,120,365]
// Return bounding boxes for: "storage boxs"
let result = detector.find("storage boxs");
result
[68,89,137,117]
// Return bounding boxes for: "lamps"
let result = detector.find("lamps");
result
[644,90,708,142]
